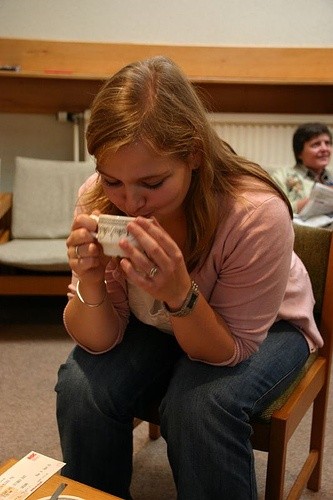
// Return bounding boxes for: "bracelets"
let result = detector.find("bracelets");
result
[76,279,107,307]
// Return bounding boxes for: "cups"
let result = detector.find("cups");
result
[88,213,154,258]
[38,494,85,500]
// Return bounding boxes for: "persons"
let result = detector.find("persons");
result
[54,56,324,500]
[268,123,333,229]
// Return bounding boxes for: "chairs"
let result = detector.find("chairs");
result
[133,221,333,500]
[0,155,101,328]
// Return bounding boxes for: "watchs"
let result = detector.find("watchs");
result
[162,281,200,319]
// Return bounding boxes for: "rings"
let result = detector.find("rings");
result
[148,265,158,280]
[75,247,80,259]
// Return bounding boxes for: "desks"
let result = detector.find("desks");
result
[0,457,125,500]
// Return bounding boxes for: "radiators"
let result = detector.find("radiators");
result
[55,107,333,174]
